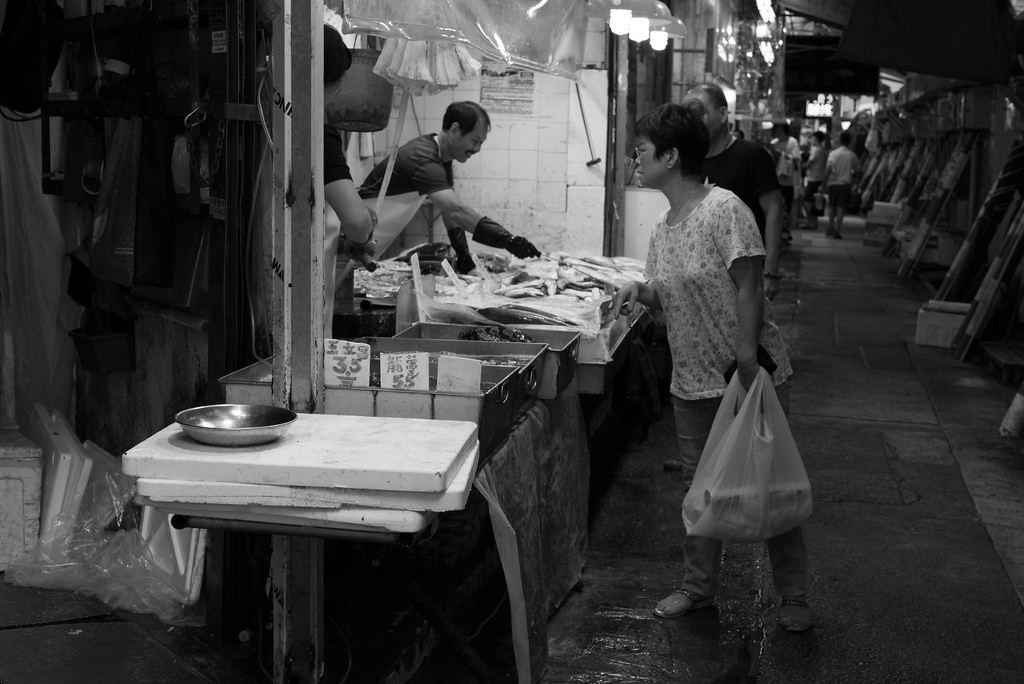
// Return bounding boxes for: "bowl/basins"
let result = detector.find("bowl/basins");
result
[68,326,134,371]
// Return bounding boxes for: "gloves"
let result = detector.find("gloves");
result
[447,227,476,275]
[473,215,540,259]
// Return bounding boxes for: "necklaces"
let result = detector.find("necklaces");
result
[669,184,699,225]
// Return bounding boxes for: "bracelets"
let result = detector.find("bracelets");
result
[764,271,783,279]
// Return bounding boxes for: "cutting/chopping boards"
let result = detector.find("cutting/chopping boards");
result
[331,297,395,338]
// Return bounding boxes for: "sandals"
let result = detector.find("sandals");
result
[778,595,812,631]
[652,589,714,617]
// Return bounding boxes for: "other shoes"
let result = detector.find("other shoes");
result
[826,229,835,235]
[834,233,842,239]
[800,222,817,230]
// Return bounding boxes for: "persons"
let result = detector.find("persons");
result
[322,25,377,338]
[683,83,786,303]
[353,101,543,275]
[610,103,811,632]
[735,122,861,240]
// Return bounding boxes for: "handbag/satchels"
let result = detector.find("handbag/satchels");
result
[814,196,826,216]
[681,363,812,541]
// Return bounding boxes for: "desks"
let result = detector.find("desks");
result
[169,373,590,684]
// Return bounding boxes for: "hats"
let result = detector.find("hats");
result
[324,22,352,83]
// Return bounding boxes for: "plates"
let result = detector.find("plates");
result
[175,404,299,444]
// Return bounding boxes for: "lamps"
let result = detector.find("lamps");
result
[584,0,687,51]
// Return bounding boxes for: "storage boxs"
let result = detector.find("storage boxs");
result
[915,308,967,348]
[861,200,901,247]
